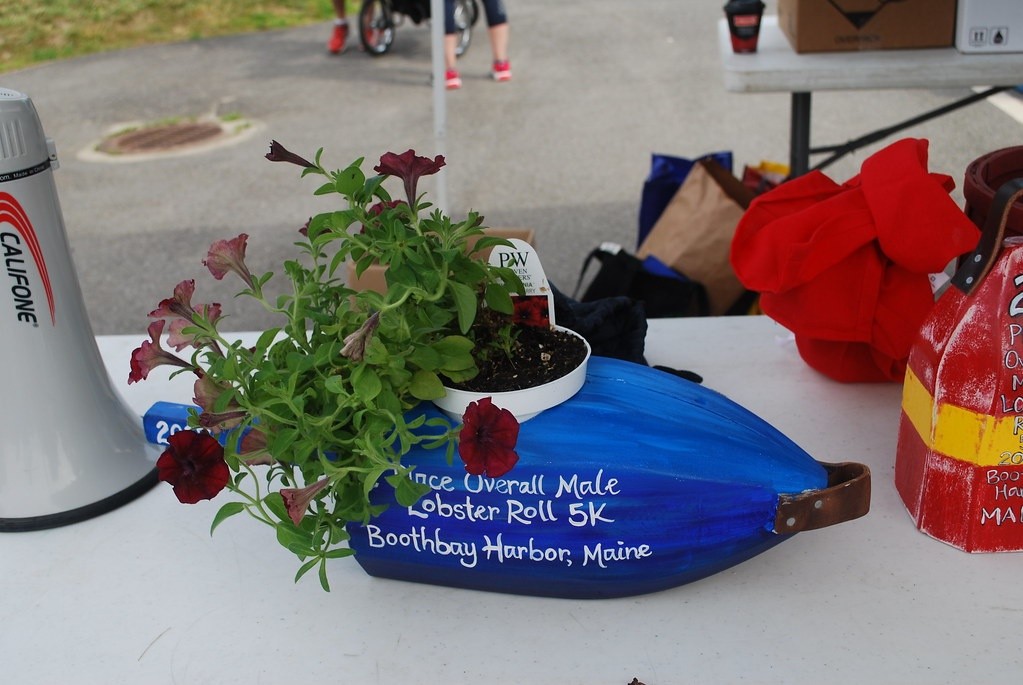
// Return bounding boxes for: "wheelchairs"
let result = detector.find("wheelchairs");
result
[358,0,478,58]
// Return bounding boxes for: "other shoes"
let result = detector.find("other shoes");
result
[359,26,377,52]
[429,70,462,89]
[492,60,511,81]
[327,24,350,54]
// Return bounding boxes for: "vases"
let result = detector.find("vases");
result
[431,322,592,428]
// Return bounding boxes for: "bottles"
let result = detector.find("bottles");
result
[894,145,1023,552]
[347,358,870,601]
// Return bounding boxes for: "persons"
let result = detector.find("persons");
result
[443,0,512,90]
[326,0,376,54]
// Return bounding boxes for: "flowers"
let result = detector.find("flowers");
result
[126,138,530,592]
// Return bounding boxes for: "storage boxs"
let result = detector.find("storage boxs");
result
[777,0,1023,56]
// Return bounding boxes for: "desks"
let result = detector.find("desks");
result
[716,13,1023,183]
[0,313,1023,685]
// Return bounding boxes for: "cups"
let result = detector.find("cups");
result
[724,0,766,54]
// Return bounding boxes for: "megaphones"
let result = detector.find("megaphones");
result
[0,88,167,534]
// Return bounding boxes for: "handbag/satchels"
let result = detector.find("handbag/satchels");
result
[572,151,791,366]
